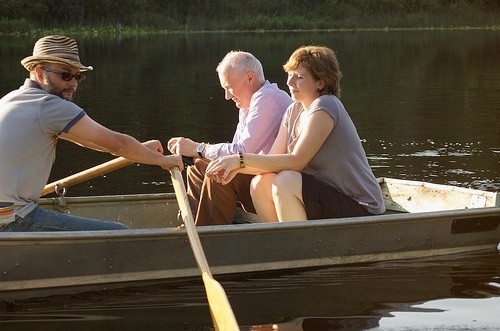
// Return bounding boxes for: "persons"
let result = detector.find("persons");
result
[167,50,297,227]
[0,34,184,230]
[206,44,387,222]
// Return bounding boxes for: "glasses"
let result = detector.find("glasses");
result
[40,67,87,83]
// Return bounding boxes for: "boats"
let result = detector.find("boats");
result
[0,177,500,303]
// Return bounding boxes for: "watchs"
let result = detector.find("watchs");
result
[197,143,205,158]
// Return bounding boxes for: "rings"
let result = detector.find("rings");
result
[220,165,224,169]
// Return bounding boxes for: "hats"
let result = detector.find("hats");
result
[21,35,93,72]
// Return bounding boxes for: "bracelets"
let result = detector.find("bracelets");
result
[238,149,244,169]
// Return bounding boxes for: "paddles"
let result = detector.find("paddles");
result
[44,156,133,196]
[171,166,241,331]
